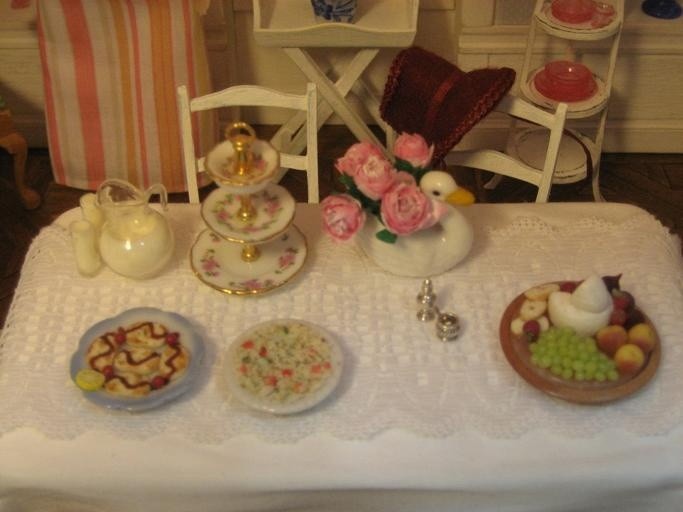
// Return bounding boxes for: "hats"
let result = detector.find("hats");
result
[380,43,516,167]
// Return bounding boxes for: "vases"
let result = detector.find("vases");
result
[365,172,475,279]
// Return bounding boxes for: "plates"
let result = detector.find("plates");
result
[67,307,205,413]
[501,280,661,403]
[551,11,593,23]
[220,319,344,415]
[535,69,598,102]
[357,202,473,278]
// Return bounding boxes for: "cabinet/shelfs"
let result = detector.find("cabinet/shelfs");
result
[481,1,625,204]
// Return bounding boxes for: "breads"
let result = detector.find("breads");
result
[87,321,189,397]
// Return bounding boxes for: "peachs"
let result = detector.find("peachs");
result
[596,323,656,375]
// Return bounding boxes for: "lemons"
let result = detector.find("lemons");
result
[77,369,106,391]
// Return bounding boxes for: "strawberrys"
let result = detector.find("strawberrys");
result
[523,320,540,341]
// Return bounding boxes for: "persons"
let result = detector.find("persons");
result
[0,95,42,211]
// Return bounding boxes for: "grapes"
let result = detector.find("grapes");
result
[529,328,618,382]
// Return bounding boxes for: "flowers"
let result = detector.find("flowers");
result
[317,133,437,244]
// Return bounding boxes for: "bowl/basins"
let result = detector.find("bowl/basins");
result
[551,1,597,16]
[546,62,592,88]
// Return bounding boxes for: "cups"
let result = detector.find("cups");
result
[591,4,616,28]
[80,193,105,226]
[69,220,101,278]
